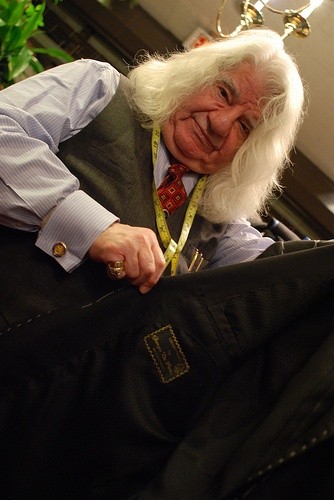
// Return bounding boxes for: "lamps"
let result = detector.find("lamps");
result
[214,0,324,42]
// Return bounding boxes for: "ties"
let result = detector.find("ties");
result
[154,157,191,221]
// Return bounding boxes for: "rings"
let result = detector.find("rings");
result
[106,259,125,277]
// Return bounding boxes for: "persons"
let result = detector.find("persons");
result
[0,24,313,332]
